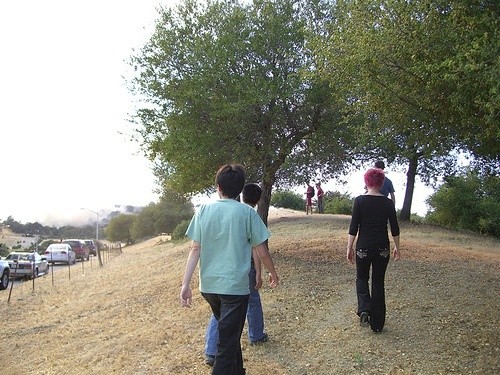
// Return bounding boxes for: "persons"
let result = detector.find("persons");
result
[304,181,314,215]
[346,169,401,334]
[180,163,279,375]
[316,183,325,214]
[364,161,396,206]
[203,183,269,365]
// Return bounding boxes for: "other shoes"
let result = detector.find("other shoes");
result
[359,312,369,327]
[373,329,382,334]
[206,356,217,367]
[250,332,268,345]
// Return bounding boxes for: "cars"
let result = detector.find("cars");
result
[0,251,49,291]
[45,243,77,264]
[61,240,97,260]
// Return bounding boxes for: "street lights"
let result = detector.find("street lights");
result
[80,208,99,240]
